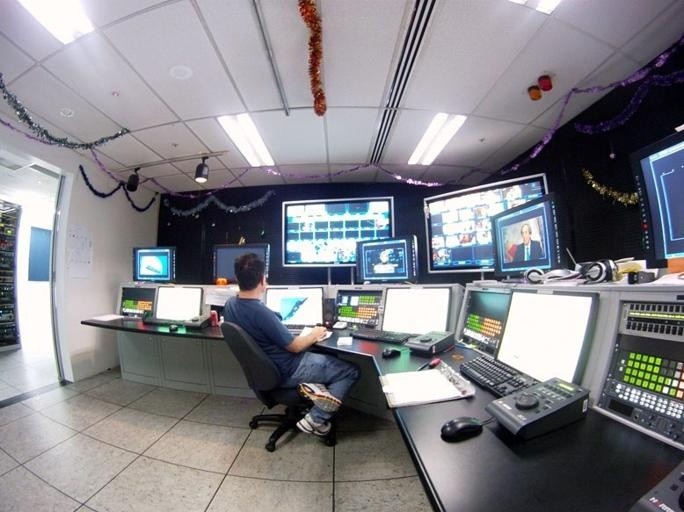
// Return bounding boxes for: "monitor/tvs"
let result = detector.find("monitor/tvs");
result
[629,130,684,269]
[495,289,600,385]
[266,288,324,325]
[116,283,159,319]
[491,191,573,277]
[334,285,383,328]
[282,196,395,268]
[460,288,511,359]
[133,246,177,282]
[214,244,270,284]
[424,173,549,274]
[356,234,420,283]
[154,286,203,321]
[378,285,452,335]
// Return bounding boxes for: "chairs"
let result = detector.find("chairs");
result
[221,321,346,452]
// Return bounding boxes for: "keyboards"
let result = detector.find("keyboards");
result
[459,354,543,398]
[143,318,185,327]
[350,327,411,344]
[288,328,304,338]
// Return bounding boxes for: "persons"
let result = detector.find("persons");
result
[223,253,361,439]
[512,223,541,263]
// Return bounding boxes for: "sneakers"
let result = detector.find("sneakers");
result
[295,414,331,436]
[296,382,342,413]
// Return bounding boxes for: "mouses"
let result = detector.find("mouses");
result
[382,348,400,359]
[441,417,483,442]
[170,325,177,331]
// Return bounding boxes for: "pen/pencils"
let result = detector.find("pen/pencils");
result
[417,362,427,370]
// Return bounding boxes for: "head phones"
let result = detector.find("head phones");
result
[579,259,619,283]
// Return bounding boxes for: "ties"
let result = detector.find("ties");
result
[526,247,530,259]
